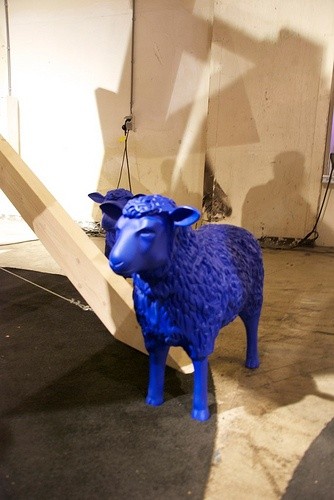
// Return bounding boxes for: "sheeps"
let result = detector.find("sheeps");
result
[89,188,146,260]
[99,194,265,420]
